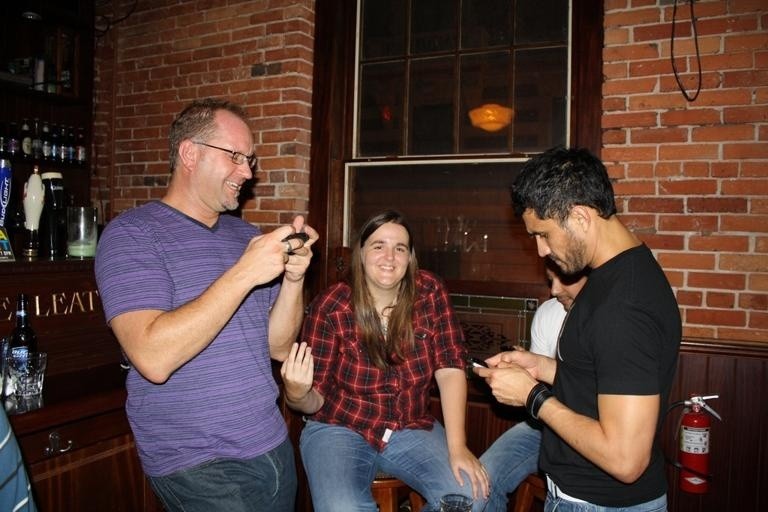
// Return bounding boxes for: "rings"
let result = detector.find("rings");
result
[285,242,291,252]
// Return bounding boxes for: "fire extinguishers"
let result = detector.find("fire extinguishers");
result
[664,394,722,494]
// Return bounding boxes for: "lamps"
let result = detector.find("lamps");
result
[470,103,515,134]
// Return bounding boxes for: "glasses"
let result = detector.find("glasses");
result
[191,142,255,168]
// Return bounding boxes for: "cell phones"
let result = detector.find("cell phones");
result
[472,358,489,369]
[282,233,310,255]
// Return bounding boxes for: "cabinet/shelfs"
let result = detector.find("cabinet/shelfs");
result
[5,389,161,512]
[1,1,96,266]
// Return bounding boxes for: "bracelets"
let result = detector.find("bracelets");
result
[525,382,553,420]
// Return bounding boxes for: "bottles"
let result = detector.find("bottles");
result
[0,113,90,166]
[67,187,79,208]
[40,211,59,259]
[22,169,46,257]
[409,213,498,281]
[44,172,65,211]
[5,291,38,413]
[60,212,68,243]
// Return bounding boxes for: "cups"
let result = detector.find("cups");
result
[67,207,97,260]
[440,494,473,512]
[8,353,47,412]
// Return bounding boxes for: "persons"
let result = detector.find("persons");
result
[478,252,594,512]
[279,212,492,512]
[93,98,320,511]
[0,402,36,512]
[473,145,683,512]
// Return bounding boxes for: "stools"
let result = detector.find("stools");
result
[514,475,548,512]
[369,479,424,512]
